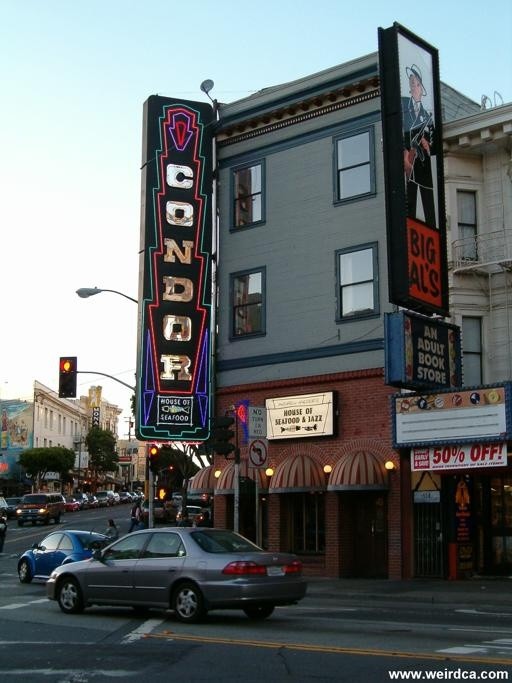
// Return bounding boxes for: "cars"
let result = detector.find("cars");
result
[141,492,213,526]
[64,484,144,511]
[0,517,7,554]
[46,526,307,624]
[16,531,110,581]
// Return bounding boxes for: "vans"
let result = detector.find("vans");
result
[17,492,64,525]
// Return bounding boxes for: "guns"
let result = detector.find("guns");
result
[404,114,433,184]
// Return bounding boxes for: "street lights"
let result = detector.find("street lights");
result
[76,287,139,305]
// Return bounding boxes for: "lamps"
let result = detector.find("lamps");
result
[265,467,274,482]
[323,464,332,478]
[384,460,398,475]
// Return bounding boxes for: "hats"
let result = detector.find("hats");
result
[405,63,428,97]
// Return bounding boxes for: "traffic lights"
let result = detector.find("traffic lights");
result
[148,444,158,463]
[59,357,76,397]
[213,416,234,454]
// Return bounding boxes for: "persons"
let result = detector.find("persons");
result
[102,516,120,542]
[127,499,143,534]
[400,64,436,228]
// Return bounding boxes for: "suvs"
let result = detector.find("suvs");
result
[5,497,20,515]
[0,497,8,519]
